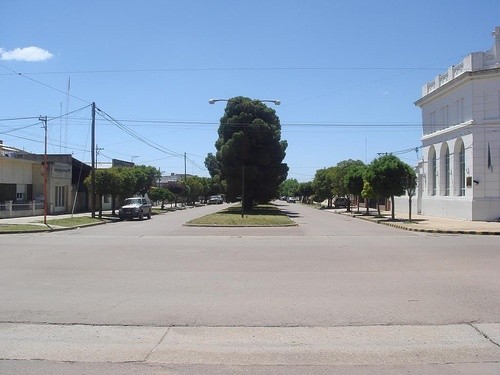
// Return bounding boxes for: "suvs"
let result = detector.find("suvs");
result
[333,197,348,208]
[119,197,152,221]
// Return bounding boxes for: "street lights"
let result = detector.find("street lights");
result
[208,98,281,219]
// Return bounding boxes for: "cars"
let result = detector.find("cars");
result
[209,196,224,204]
[320,200,335,208]
[287,197,296,203]
